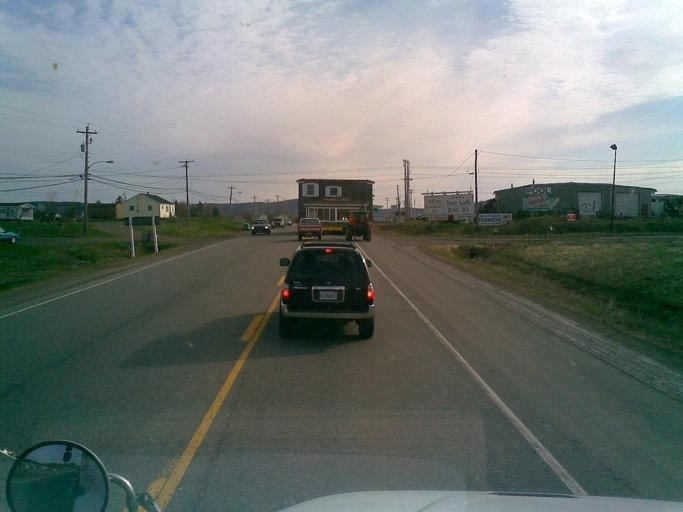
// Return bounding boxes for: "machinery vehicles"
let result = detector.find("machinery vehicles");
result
[343,209,373,241]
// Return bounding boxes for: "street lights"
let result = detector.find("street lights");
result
[83,159,115,234]
[608,143,618,233]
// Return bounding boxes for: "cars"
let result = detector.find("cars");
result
[271,216,286,229]
[0,227,22,245]
[296,217,323,242]
[250,219,272,235]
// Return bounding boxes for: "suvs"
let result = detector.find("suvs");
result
[278,236,377,343]
